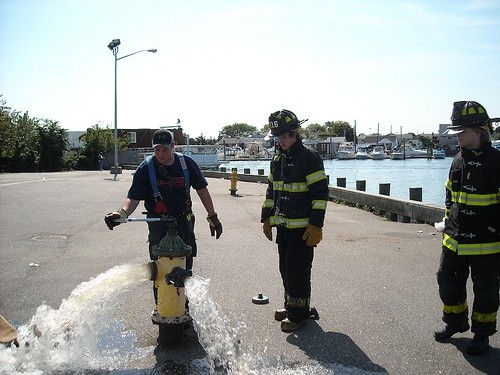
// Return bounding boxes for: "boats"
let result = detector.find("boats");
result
[392,146,403,159]
[337,142,355,160]
[354,144,372,159]
[433,148,445,159]
[372,145,387,160]
[173,145,222,170]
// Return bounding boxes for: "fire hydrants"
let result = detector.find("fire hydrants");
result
[145,220,207,362]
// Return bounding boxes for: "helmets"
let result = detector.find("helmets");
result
[264,109,308,140]
[442,101,500,136]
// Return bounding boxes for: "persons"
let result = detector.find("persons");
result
[260,109,329,330]
[105,129,222,304]
[433,102,500,352]
[98,152,106,170]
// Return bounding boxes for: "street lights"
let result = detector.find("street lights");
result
[107,38,158,181]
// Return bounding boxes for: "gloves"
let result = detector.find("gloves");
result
[105,208,128,230]
[263,218,272,241]
[302,224,323,246]
[207,212,222,239]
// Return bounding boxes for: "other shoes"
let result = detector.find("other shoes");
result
[465,335,489,356]
[280,317,308,332]
[275,308,288,321]
[433,321,469,341]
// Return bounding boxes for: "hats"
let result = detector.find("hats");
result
[153,129,174,148]
